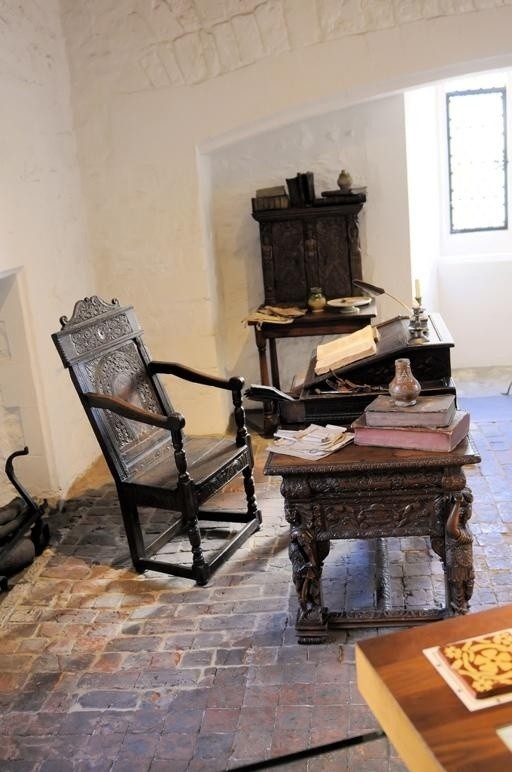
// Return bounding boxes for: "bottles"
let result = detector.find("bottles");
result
[337,169,353,191]
[308,287,326,313]
[388,358,421,409]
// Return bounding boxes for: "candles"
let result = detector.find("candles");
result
[415,277,422,298]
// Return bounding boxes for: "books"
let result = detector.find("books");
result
[314,325,377,376]
[365,395,457,428]
[438,628,511,698]
[351,408,470,454]
[373,326,380,344]
[421,629,512,711]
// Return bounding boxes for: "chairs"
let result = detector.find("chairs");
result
[52,293,264,586]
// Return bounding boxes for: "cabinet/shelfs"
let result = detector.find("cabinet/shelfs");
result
[261,216,361,307]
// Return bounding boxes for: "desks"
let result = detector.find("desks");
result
[250,299,377,389]
[266,411,482,645]
[351,601,511,772]
